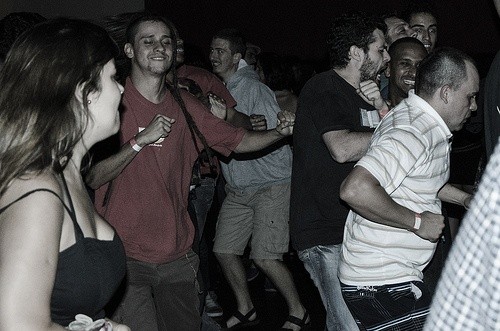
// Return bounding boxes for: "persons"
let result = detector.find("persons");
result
[84,12,296,331]
[0,19,129,331]
[164,7,500,331]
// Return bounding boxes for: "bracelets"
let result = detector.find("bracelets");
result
[410,213,422,233]
[378,106,388,120]
[129,139,142,153]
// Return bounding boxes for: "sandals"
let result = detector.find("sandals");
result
[279,310,311,331]
[217,306,259,331]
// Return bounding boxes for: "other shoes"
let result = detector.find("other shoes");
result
[265,282,278,292]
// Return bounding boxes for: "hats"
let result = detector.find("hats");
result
[246,39,262,55]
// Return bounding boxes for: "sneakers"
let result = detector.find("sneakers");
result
[246,259,260,281]
[202,291,224,317]
